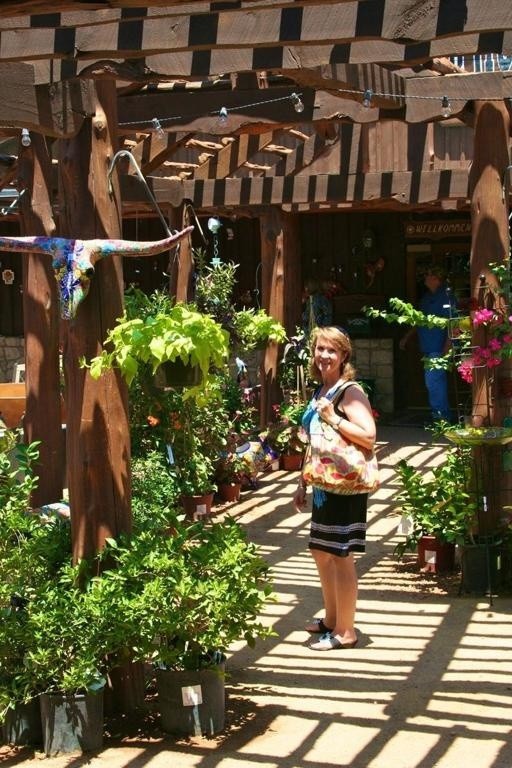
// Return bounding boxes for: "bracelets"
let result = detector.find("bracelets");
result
[297,484,309,492]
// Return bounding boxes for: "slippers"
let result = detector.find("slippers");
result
[307,631,358,652]
[304,618,333,634]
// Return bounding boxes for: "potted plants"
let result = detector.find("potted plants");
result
[24,559,137,757]
[129,450,175,537]
[210,448,250,500]
[390,445,512,597]
[1,417,69,748]
[176,455,218,518]
[269,399,314,471]
[70,540,147,726]
[78,306,230,408]
[92,525,279,736]
[189,403,257,455]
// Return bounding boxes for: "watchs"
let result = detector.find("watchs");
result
[333,417,345,432]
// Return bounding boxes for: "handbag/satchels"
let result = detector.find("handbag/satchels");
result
[302,380,381,496]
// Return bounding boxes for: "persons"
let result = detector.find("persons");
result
[292,326,383,651]
[398,271,455,426]
